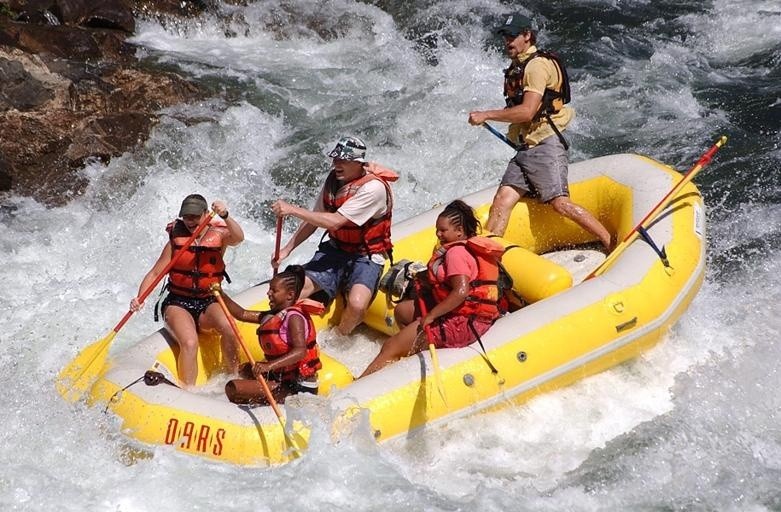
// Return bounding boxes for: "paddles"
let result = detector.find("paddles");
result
[211,285,308,460]
[414,278,455,414]
[56,211,217,403]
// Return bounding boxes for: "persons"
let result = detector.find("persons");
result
[270,136,402,338]
[466,13,615,254]
[207,266,324,408]
[129,192,244,388]
[354,198,503,381]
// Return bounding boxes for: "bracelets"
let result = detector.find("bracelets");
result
[220,211,229,219]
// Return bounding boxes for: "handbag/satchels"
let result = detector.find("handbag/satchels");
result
[380,260,424,303]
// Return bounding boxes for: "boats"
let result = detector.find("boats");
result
[90,153,705,467]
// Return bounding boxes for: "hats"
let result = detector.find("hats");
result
[497,14,530,33]
[329,137,367,163]
[179,199,207,217]
